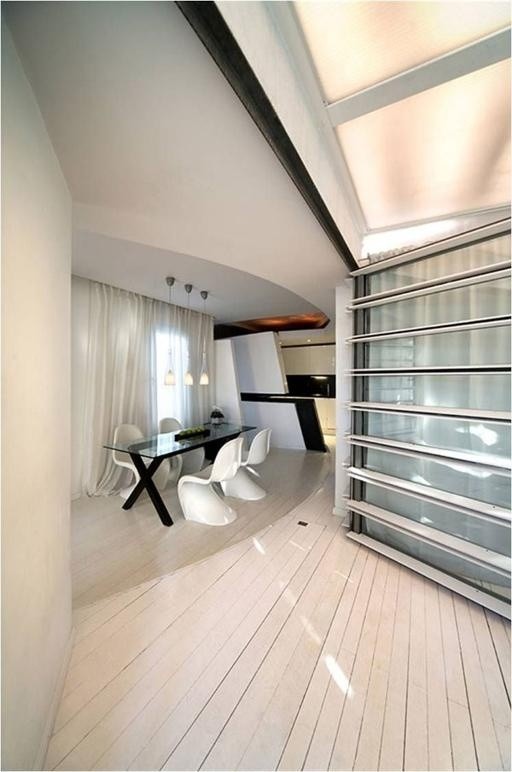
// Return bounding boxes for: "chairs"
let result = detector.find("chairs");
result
[113,418,271,526]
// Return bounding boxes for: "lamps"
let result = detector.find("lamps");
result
[162,277,210,385]
